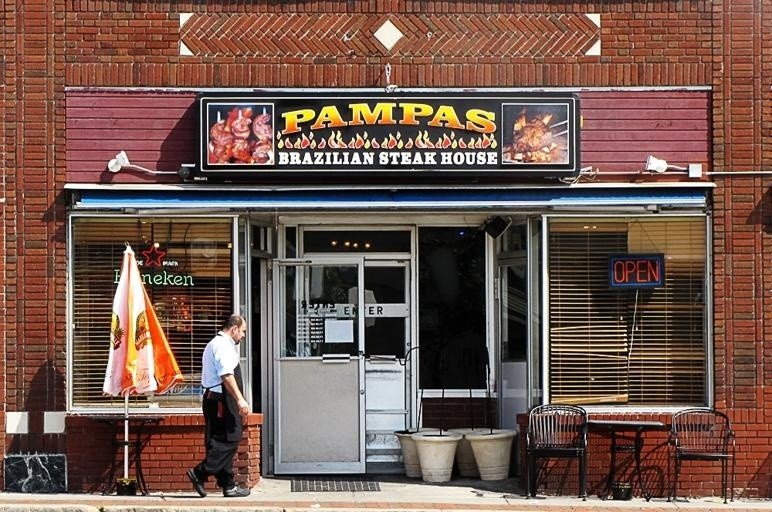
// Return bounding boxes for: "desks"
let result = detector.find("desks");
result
[576,415,671,503]
[87,410,166,498]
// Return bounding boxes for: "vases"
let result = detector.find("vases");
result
[390,420,519,487]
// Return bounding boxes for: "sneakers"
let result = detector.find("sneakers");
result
[223,486,250,497]
[187,469,207,497]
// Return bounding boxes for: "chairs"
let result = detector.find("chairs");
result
[666,407,737,505]
[520,402,589,501]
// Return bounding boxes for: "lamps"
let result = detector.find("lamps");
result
[107,150,130,173]
[643,152,669,176]
[481,213,514,242]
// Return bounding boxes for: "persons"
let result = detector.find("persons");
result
[186,314,250,497]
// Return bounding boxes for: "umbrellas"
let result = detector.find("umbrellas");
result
[101,246,184,479]
